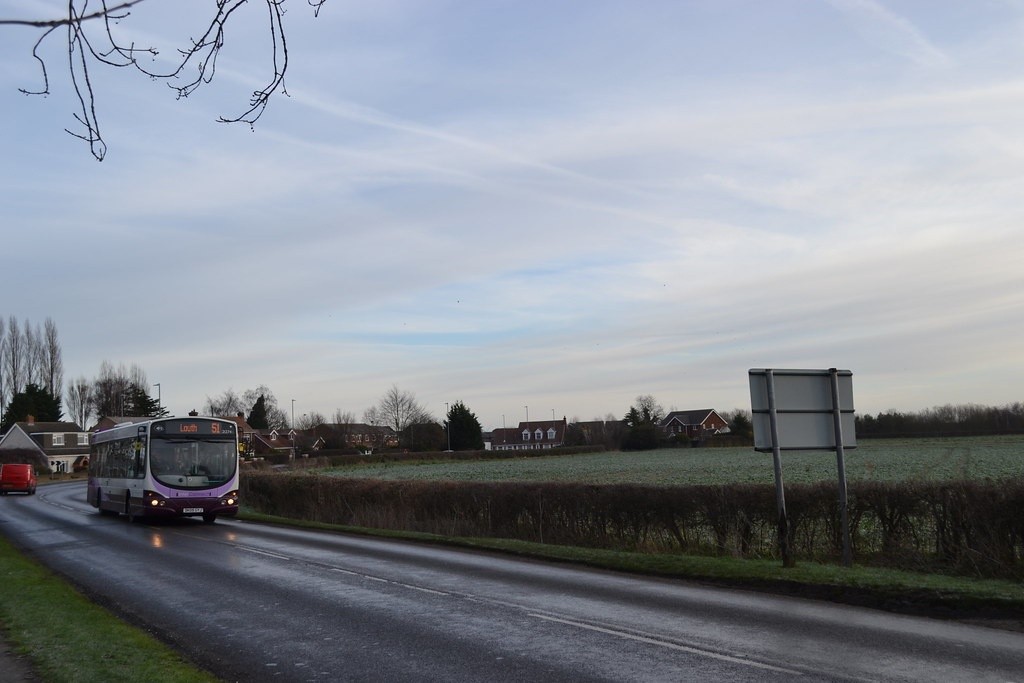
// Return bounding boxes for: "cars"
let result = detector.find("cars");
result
[0,464,38,496]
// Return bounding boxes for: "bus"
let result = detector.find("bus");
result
[86,416,248,526]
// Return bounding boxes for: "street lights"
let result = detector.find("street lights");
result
[446,403,449,450]
[291,399,297,460]
[154,383,162,419]
[525,405,529,446]
[503,414,506,445]
[552,409,555,445]
